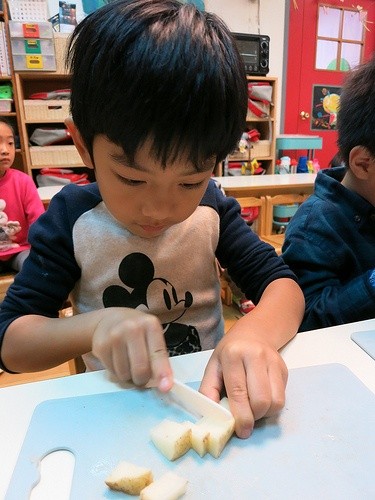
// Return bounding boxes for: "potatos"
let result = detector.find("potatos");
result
[103,395,235,500]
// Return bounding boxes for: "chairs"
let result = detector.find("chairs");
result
[0,193,313,378]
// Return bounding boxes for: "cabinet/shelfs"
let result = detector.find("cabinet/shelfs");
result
[0,0,278,177]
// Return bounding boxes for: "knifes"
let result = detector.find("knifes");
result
[166,381,235,431]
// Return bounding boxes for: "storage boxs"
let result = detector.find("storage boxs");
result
[29,144,84,165]
[7,20,57,71]
[246,102,270,120]
[23,99,71,121]
[229,140,269,160]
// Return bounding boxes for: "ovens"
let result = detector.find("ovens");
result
[230,32,270,76]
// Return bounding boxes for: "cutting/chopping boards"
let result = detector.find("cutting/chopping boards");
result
[5,362,375,500]
[350,329,375,361]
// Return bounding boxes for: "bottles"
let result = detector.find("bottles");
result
[275,155,320,175]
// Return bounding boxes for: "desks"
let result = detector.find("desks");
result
[37,173,317,200]
[0,318,375,500]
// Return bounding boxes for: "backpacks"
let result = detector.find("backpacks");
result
[241,207,258,225]
[241,129,261,143]
[34,169,88,186]
[29,130,70,145]
[228,159,267,175]
[248,81,273,118]
[25,89,72,101]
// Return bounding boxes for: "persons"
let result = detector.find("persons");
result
[281,57,375,333]
[0,0,307,439]
[0,119,46,273]
